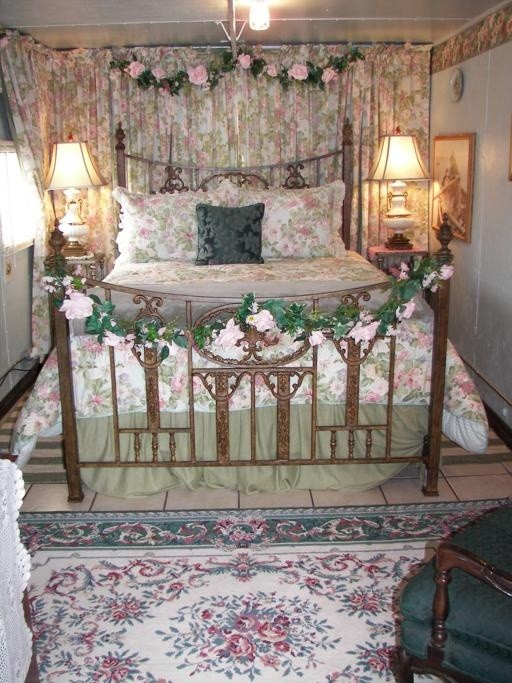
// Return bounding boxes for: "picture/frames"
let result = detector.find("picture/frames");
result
[432,131,478,244]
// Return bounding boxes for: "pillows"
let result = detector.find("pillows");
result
[110,178,223,264]
[224,178,347,260]
[194,201,267,266]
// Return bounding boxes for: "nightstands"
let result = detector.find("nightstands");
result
[366,245,427,279]
[62,251,106,290]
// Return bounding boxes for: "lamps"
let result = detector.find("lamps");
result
[363,132,433,251]
[43,139,110,256]
[248,0,271,31]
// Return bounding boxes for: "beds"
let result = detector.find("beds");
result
[9,117,493,504]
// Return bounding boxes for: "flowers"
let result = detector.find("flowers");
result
[39,253,455,360]
[107,41,367,99]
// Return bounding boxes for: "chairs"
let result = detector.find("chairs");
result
[385,498,512,681]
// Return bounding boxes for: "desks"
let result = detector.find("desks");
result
[0,447,42,682]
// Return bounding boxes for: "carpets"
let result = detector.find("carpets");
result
[0,377,512,485]
[15,497,511,681]
